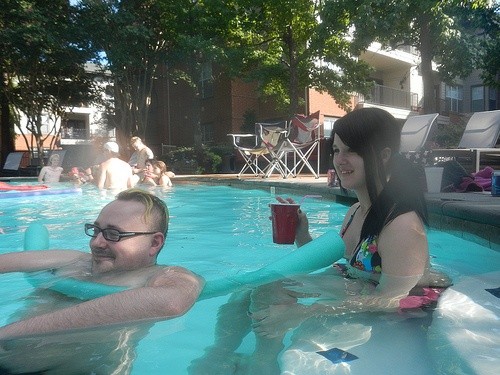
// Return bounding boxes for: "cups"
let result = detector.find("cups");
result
[424,166,443,193]
[270,204,301,245]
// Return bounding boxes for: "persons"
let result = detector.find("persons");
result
[69,163,99,186]
[146,161,173,188]
[184,105,457,277]
[96,141,136,190]
[128,135,154,180]
[38,154,64,184]
[138,158,175,188]
[84,188,170,275]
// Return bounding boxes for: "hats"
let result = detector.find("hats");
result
[104,142,119,153]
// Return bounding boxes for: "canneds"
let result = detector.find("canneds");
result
[327,168,335,184]
[491,170,500,197]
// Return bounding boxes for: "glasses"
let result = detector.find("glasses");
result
[85,223,156,242]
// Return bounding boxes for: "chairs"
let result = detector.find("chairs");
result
[433,109,500,173]
[226,121,288,179]
[36,149,66,175]
[267,110,321,179]
[15,150,32,176]
[1,152,24,177]
[400,112,439,153]
